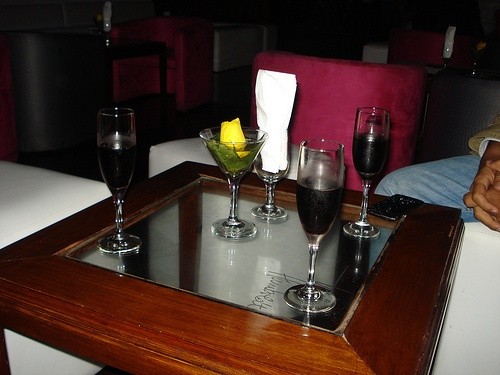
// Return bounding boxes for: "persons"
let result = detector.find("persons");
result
[369,117,500,275]
[396,0,424,30]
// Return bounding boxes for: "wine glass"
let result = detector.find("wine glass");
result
[284,138,345,313]
[471,44,485,67]
[96,107,143,254]
[343,108,390,239]
[251,140,291,221]
[102,20,112,42]
[199,126,269,240]
[92,17,104,34]
[441,48,452,70]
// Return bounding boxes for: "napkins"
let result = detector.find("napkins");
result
[254,69,297,173]
[443,23,458,58]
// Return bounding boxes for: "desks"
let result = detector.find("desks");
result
[1,158,464,375]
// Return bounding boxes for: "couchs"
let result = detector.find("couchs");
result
[0,17,500,374]
